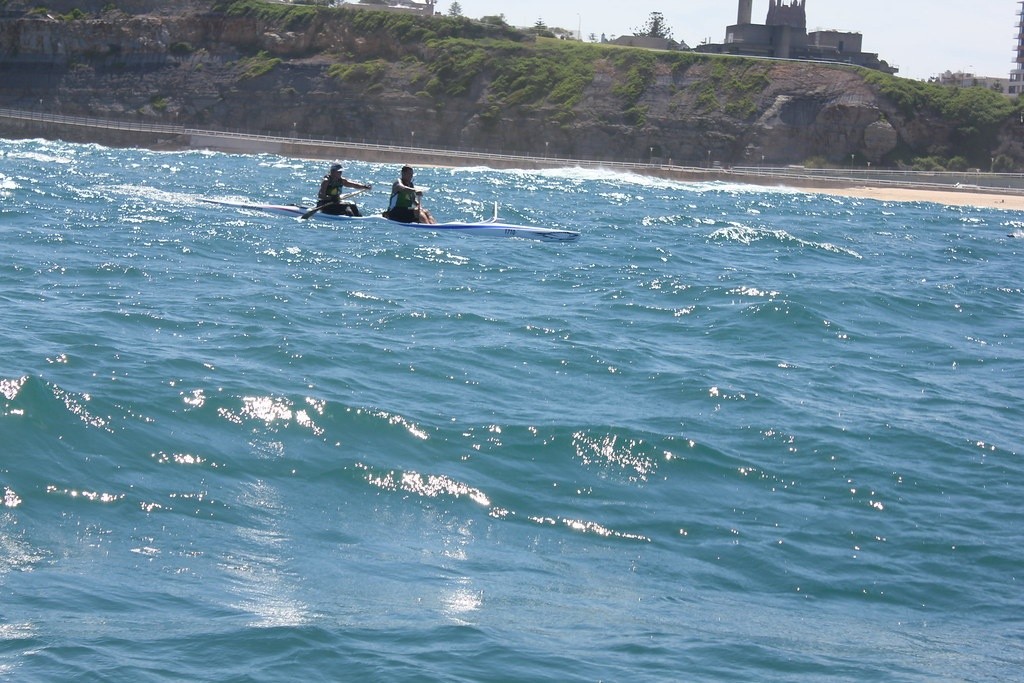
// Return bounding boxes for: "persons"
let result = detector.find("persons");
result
[388,166,435,224]
[317,163,372,216]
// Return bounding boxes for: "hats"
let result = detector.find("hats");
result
[330,163,344,172]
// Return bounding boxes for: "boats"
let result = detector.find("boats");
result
[195,193,580,244]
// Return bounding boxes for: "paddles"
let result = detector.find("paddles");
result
[417,196,421,214]
[300,188,367,219]
